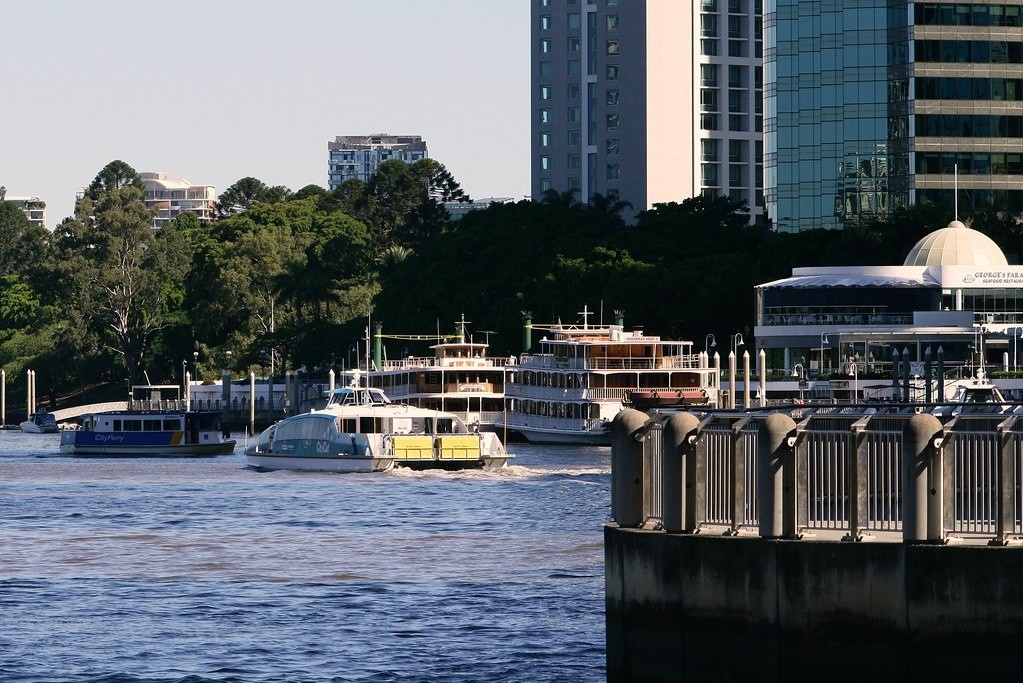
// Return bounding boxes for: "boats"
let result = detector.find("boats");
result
[242,325,516,474]
[339,300,723,444]
[20,406,60,433]
[58,409,237,459]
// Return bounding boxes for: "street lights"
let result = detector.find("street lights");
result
[227,351,232,366]
[820,331,830,374]
[123,378,129,410]
[848,363,858,405]
[914,374,920,404]
[729,334,741,352]
[193,351,198,385]
[790,363,806,399]
[348,343,356,370]
[734,332,746,374]
[705,333,716,354]
